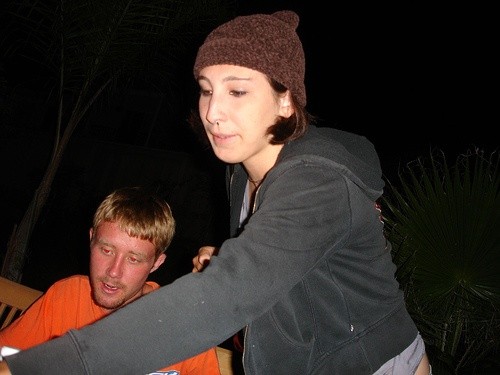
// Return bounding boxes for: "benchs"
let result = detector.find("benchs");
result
[1,277,239,375]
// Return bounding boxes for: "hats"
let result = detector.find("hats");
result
[193,9,307,106]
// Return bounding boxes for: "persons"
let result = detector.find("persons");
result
[0,182,224,375]
[1,7,430,373]
